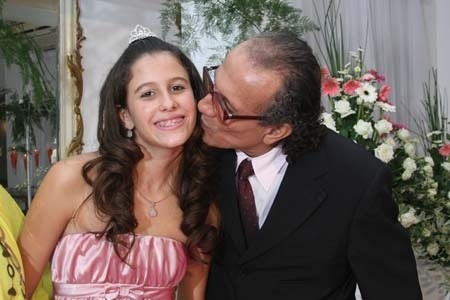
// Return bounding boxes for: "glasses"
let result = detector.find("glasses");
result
[203,65,272,123]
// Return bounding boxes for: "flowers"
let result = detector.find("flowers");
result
[314,1,450,299]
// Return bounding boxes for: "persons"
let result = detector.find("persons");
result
[198,31,423,300]
[16,37,222,300]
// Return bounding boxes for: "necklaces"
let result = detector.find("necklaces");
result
[135,190,174,217]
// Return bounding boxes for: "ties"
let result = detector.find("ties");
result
[237,160,259,239]
